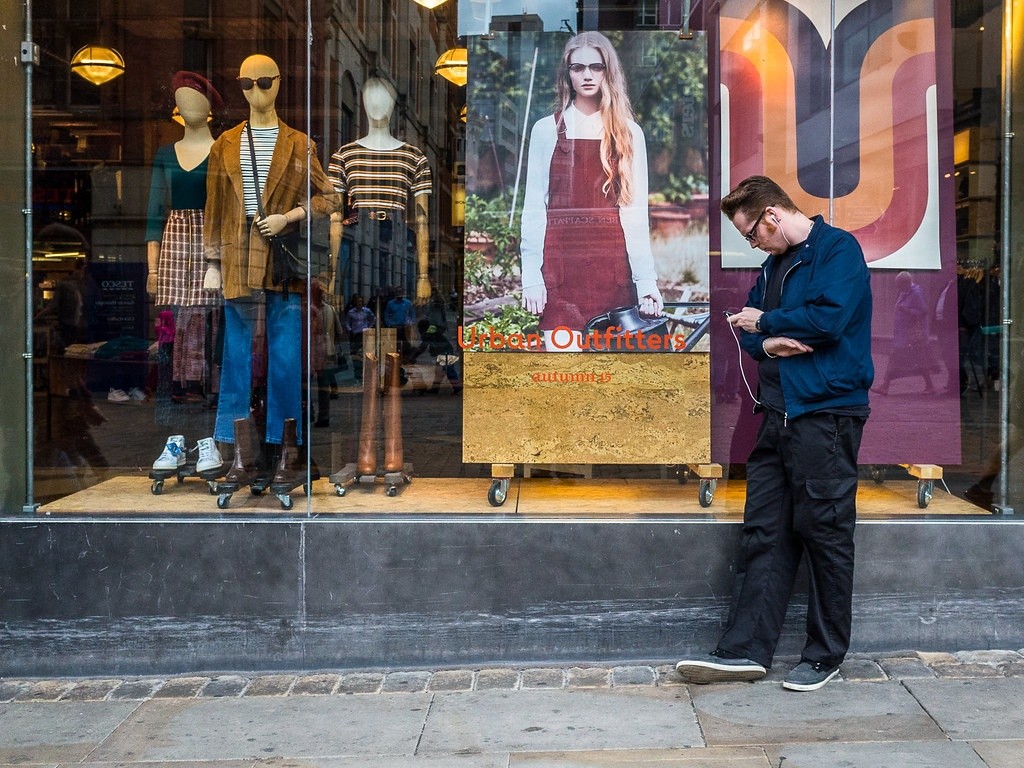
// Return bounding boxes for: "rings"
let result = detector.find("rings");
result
[786,338,794,341]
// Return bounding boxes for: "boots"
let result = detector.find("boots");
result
[356,351,378,476]
[384,352,403,472]
[225,418,257,482]
[273,418,298,483]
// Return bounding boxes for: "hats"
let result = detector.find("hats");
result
[171,71,225,111]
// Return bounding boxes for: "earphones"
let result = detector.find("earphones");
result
[771,214,779,224]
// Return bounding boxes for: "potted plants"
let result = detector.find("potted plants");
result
[649,173,709,240]
[465,193,548,351]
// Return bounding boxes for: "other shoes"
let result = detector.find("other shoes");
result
[314,419,330,428]
[424,388,439,395]
[452,385,465,396]
[871,385,890,398]
[922,386,938,396]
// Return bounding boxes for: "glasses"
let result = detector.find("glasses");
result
[568,62,607,72]
[237,75,281,90]
[746,202,776,244]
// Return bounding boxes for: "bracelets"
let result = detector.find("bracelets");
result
[755,312,764,333]
[762,337,778,358]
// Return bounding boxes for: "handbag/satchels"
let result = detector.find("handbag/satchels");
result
[888,347,939,378]
[333,353,348,371]
[271,230,330,298]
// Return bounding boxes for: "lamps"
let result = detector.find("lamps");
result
[69,0,126,86]
[171,106,212,126]
[434,38,467,87]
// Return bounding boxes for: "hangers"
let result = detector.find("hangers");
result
[957,258,1000,283]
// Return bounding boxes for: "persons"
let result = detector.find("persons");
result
[155,279,343,430]
[32,223,147,507]
[203,55,340,484]
[327,77,430,474]
[520,31,664,351]
[145,71,225,472]
[964,341,1024,514]
[676,176,875,691]
[871,270,936,396]
[929,264,980,398]
[343,285,463,396]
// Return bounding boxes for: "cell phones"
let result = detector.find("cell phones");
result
[724,310,734,317]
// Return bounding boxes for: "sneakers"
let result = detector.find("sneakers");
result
[197,438,223,471]
[108,388,130,404]
[128,387,149,403]
[784,651,841,691]
[675,652,766,685]
[155,436,188,469]
